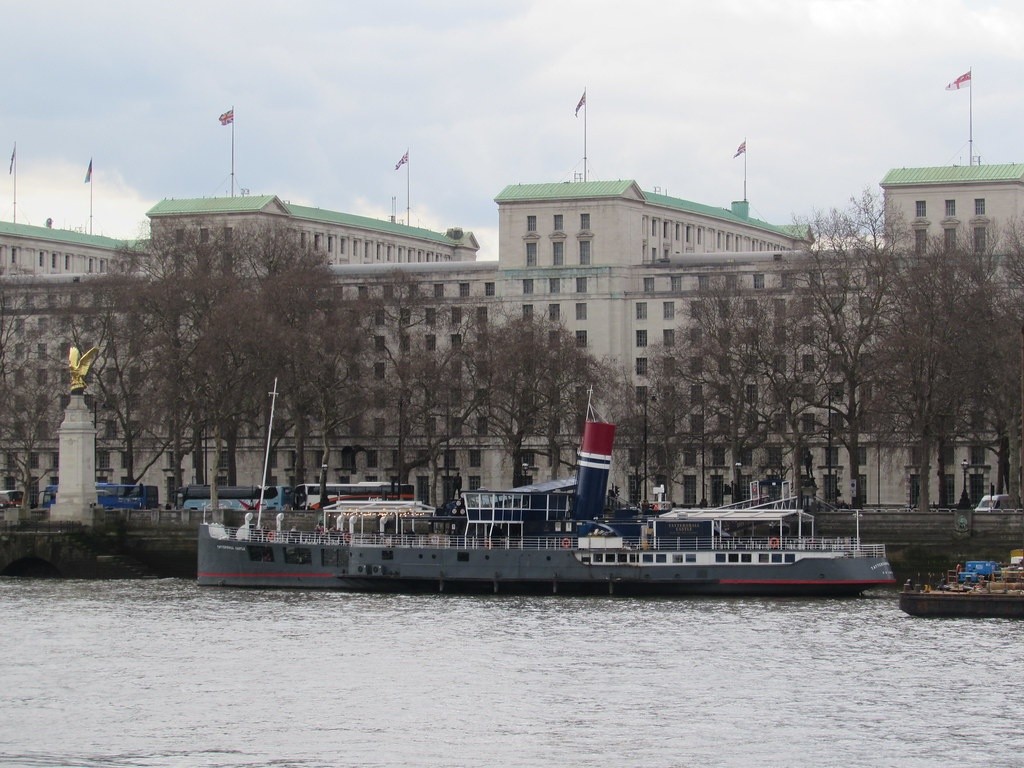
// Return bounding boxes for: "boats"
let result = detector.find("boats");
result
[898,565,1024,618]
[195,377,899,598]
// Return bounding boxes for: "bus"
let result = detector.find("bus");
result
[289,483,414,510]
[0,488,25,508]
[35,484,159,511]
[169,484,292,512]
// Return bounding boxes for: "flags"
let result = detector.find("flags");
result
[945,71,970,90]
[733,142,745,158]
[219,110,233,125]
[85,161,91,183]
[394,151,407,171]
[9,146,15,174]
[575,92,585,118]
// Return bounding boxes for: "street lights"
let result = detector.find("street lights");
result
[955,458,972,509]
[734,460,742,504]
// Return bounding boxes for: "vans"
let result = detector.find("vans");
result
[974,492,1011,514]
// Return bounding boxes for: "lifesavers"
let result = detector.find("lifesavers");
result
[770,538,780,547]
[563,538,571,548]
[268,532,275,540]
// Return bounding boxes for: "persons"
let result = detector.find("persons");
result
[452,472,462,500]
[314,520,349,535]
[804,451,814,479]
